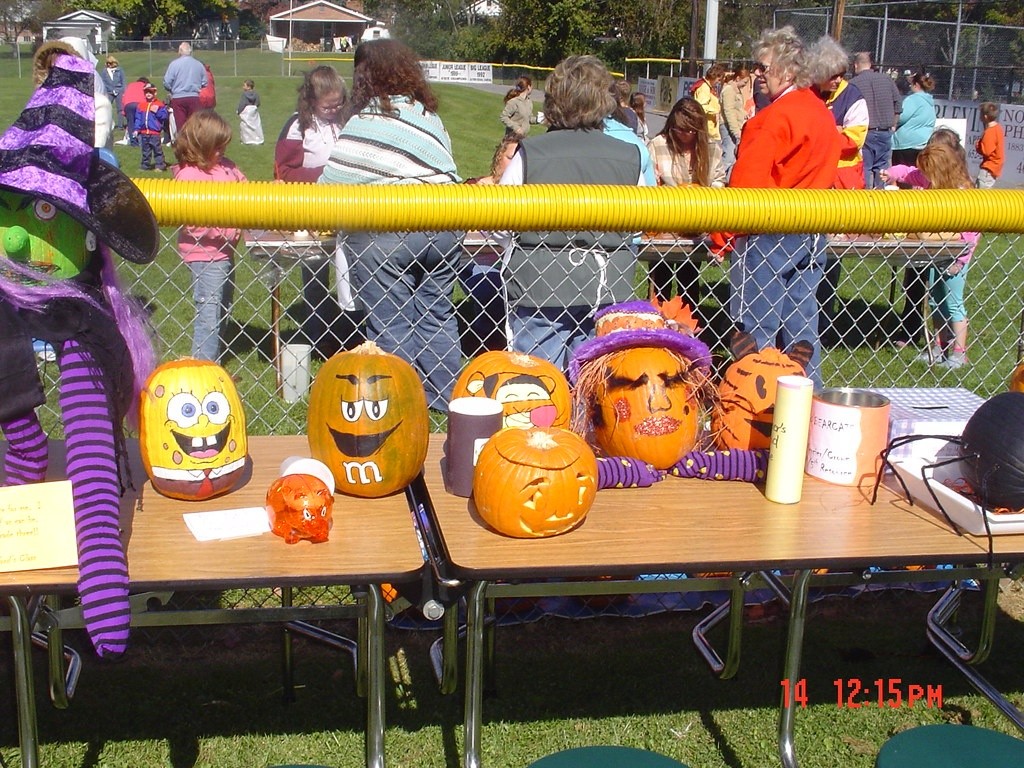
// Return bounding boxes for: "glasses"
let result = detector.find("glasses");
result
[753,64,770,72]
[321,105,343,114]
[673,129,696,136]
[830,71,846,80]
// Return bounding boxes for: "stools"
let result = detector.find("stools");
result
[526,745,687,768]
[876,725,1024,768]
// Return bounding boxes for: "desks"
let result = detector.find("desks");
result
[246,232,975,399]
[1,429,1024,768]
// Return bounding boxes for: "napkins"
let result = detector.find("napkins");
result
[182,507,271,541]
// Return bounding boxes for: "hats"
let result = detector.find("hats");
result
[903,70,911,75]
[143,83,156,90]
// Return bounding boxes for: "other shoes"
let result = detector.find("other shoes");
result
[895,332,920,348]
[319,339,335,361]
[914,348,964,369]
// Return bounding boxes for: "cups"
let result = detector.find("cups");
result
[447,397,503,498]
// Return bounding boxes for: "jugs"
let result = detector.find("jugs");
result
[280,344,311,399]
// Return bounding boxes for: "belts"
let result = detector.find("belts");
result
[868,128,889,131]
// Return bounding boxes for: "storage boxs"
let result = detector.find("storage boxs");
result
[858,387,986,475]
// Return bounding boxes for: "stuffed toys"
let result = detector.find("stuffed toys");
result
[0,39,158,660]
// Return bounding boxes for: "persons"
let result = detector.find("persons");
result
[642,97,726,334]
[880,128,982,369]
[975,102,1005,190]
[491,55,657,386]
[890,72,936,189]
[235,80,264,146]
[473,132,525,185]
[801,34,904,190]
[170,110,250,361]
[273,64,350,358]
[691,61,775,187]
[98,38,219,174]
[318,39,466,412]
[708,26,865,393]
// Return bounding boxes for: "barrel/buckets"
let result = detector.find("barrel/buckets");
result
[806,388,891,488]
